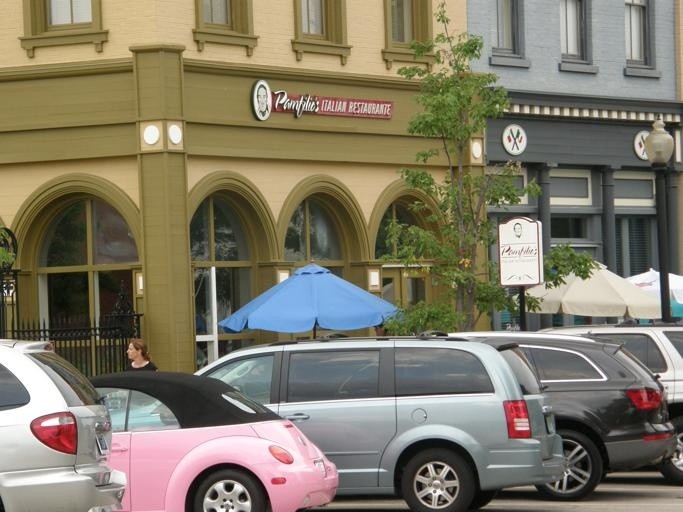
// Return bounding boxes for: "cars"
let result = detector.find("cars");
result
[85,372,338,512]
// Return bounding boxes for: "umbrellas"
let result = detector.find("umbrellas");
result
[511,259,672,325]
[624,268,682,307]
[215,259,401,339]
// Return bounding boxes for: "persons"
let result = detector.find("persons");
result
[256,85,269,118]
[125,339,157,371]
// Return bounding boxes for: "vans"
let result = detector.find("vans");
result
[191,328,567,511]
[0,339,124,512]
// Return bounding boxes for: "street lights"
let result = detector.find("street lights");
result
[646,112,675,322]
